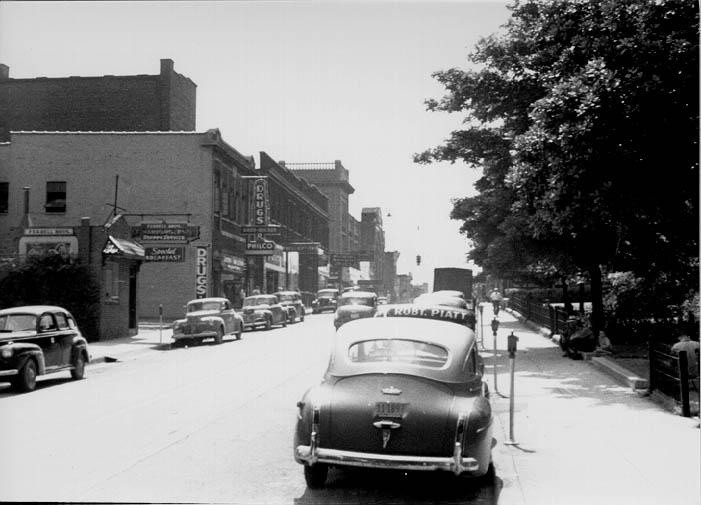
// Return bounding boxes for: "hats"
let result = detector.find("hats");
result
[566,315,577,323]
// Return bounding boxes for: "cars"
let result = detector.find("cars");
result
[0,305,93,393]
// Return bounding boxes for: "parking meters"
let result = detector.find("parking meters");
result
[506,331,522,446]
[159,303,164,346]
[489,316,500,391]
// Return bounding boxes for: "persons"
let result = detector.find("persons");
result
[559,310,613,360]
[491,287,502,315]
[669,329,700,379]
